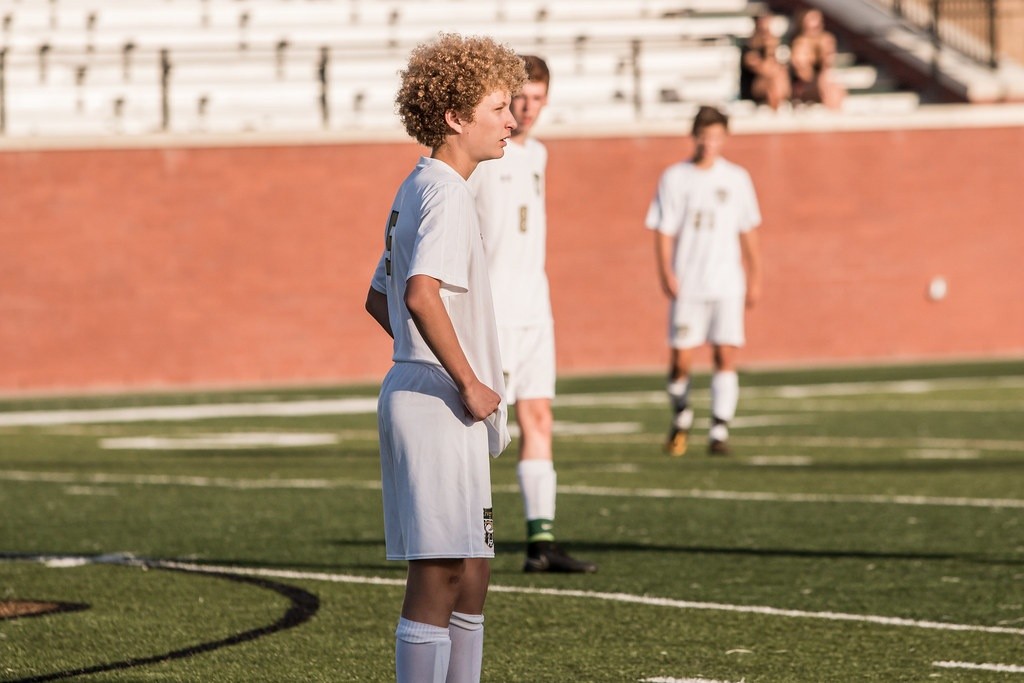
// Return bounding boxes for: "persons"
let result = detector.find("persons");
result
[643,105,764,458]
[364,36,510,683]
[739,8,840,112]
[464,55,598,577]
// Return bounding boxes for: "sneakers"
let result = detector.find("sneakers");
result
[662,420,693,458]
[705,434,737,458]
[521,540,600,575]
